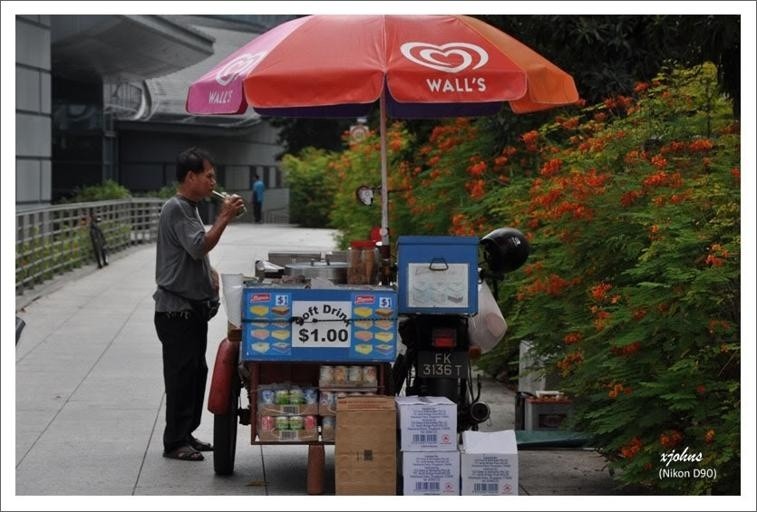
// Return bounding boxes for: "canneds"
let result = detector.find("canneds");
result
[255,363,378,433]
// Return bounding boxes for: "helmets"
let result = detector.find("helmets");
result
[480,227,529,273]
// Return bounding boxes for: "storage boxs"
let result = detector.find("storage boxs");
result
[525,396,573,432]
[335,395,519,495]
[397,235,478,315]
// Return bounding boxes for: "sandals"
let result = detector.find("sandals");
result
[163,439,214,460]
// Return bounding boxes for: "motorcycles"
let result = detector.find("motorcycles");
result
[383,246,504,430]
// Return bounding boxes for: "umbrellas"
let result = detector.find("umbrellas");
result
[184,15,586,244]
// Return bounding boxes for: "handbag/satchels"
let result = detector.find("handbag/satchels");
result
[190,296,220,321]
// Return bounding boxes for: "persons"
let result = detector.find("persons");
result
[152,147,244,463]
[251,173,265,223]
